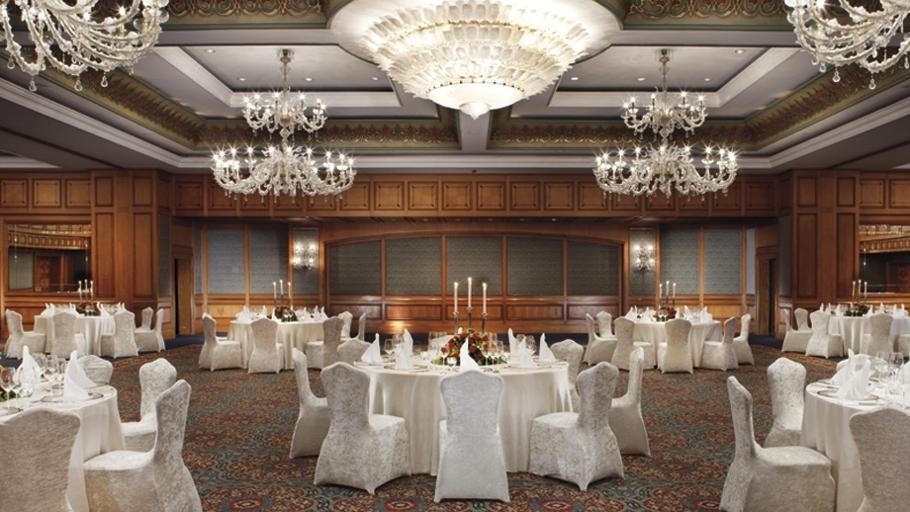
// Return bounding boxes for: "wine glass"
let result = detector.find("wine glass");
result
[12,371,24,413]
[55,358,66,401]
[516,334,525,344]
[496,341,503,370]
[0,367,16,413]
[44,355,58,401]
[428,331,456,375]
[875,352,890,386]
[385,339,392,369]
[32,353,47,404]
[392,334,403,371]
[526,336,536,356]
[482,340,491,372]
[889,352,904,387]
[489,340,497,372]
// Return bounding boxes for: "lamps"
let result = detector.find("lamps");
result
[785,1,910,89]
[359,0,592,118]
[210,49,356,202]
[0,0,171,91]
[593,47,739,202]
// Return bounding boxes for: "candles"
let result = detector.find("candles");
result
[666,281,669,296]
[454,282,458,313]
[482,283,487,314]
[858,280,861,294]
[273,281,276,299]
[853,282,855,296]
[85,280,87,291]
[90,281,94,295]
[659,284,663,299]
[280,280,283,295]
[288,282,290,297]
[864,282,868,296]
[467,277,472,308]
[79,281,81,292]
[673,283,676,298]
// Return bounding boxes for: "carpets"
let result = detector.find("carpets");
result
[1,344,909,512]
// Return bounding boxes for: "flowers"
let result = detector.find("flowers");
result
[0,365,20,402]
[441,330,498,365]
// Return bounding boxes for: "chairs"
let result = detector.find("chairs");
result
[429,335,454,349]
[850,408,910,512]
[657,319,694,375]
[201,313,227,341]
[0,409,80,512]
[248,318,284,375]
[702,316,739,372]
[136,307,153,331]
[894,335,909,359]
[337,339,371,364]
[6,311,46,360]
[199,315,242,372]
[338,311,353,338]
[609,347,652,458]
[765,357,806,447]
[861,314,893,357]
[314,361,412,495]
[51,312,87,360]
[596,310,616,338]
[551,339,584,412]
[121,359,177,451]
[733,313,754,365]
[583,313,618,366]
[4,310,11,356]
[805,310,843,359]
[781,309,813,353]
[528,361,624,492]
[721,375,834,511]
[101,311,138,359]
[289,349,332,459]
[134,308,166,353]
[611,317,654,370]
[83,379,203,512]
[434,370,511,503]
[340,312,366,344]
[65,354,113,384]
[305,316,344,371]
[795,308,808,329]
